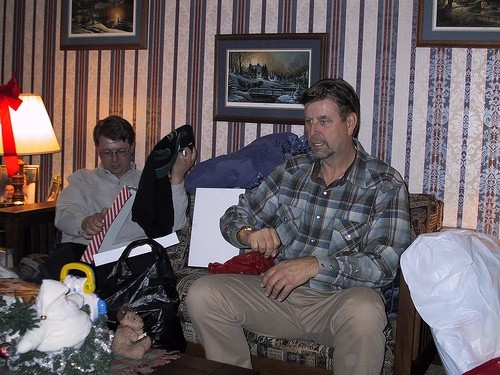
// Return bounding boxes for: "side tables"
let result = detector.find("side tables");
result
[0,201,57,266]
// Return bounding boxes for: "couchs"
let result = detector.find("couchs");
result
[166,194,444,375]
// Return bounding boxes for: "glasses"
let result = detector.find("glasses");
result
[97,144,130,158]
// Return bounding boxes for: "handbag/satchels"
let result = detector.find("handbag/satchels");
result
[99,238,186,353]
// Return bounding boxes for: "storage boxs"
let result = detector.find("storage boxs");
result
[80,184,179,266]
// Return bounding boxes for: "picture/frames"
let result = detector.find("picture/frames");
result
[213,33,329,125]
[0,164,40,204]
[416,0,500,47]
[60,0,148,50]
[46,181,60,202]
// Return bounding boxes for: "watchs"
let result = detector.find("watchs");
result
[237,226,260,246]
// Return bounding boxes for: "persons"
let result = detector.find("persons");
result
[2,172,36,204]
[188,77,411,375]
[51,113,197,293]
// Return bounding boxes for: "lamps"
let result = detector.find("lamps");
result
[0,94,61,206]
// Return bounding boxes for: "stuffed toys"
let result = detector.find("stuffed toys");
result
[112,306,152,359]
